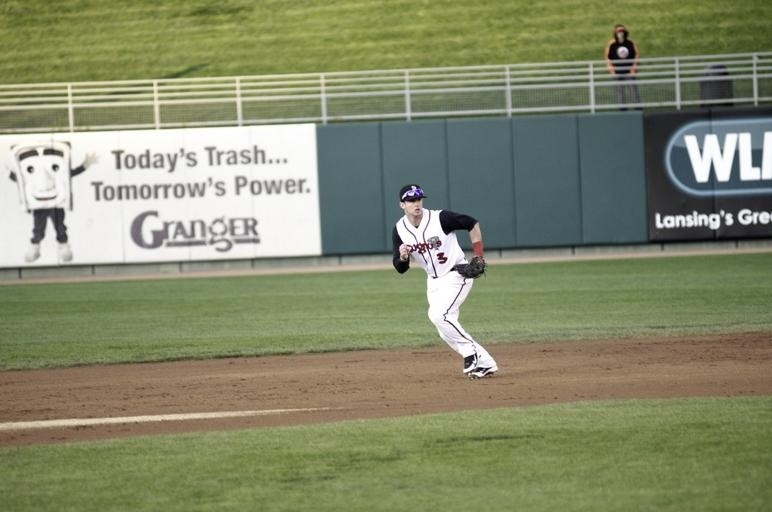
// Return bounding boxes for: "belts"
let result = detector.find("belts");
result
[451,268,456,272]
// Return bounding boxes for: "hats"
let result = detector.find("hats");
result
[399,184,428,201]
[616,26,625,33]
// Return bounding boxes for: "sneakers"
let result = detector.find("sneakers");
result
[468,365,498,379]
[463,346,478,374]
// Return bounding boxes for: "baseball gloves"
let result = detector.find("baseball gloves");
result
[456,256,484,278]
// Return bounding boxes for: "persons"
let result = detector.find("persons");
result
[392,184,499,378]
[604,25,643,110]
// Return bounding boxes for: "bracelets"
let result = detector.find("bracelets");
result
[472,242,483,257]
